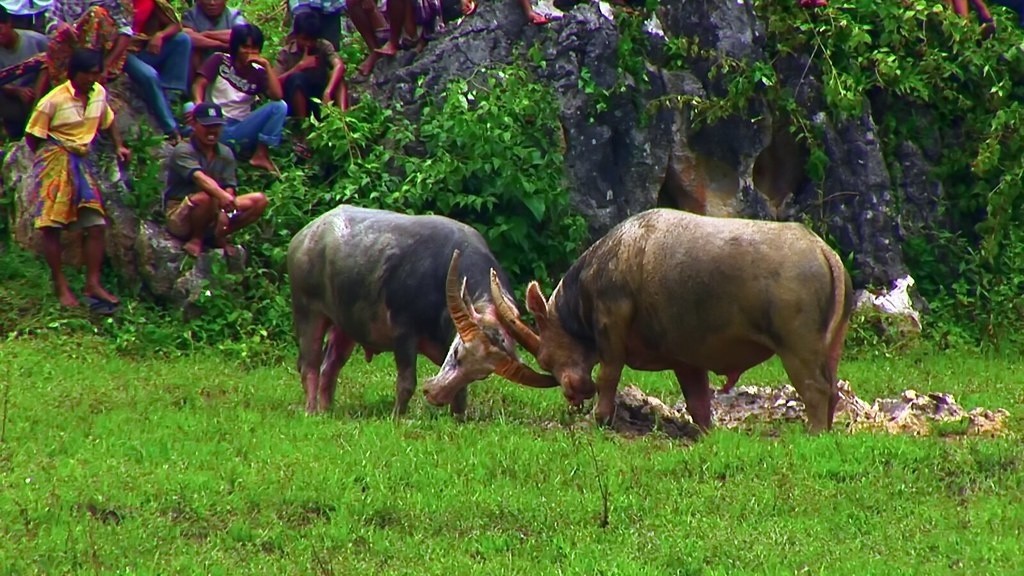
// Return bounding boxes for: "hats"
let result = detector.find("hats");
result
[192,103,228,125]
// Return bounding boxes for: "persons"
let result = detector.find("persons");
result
[520,0,564,24]
[273,12,348,159]
[164,103,270,258]
[24,46,131,308]
[799,0,997,42]
[279,0,477,76]
[181,24,288,176]
[0,0,248,145]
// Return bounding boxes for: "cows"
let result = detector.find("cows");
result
[285,199,564,425]
[484,205,861,441]
